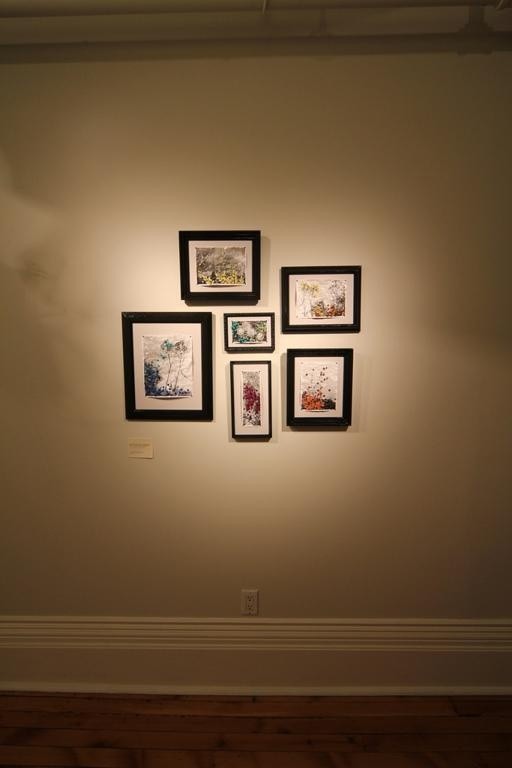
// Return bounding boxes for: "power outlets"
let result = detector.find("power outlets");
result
[242,591,258,616]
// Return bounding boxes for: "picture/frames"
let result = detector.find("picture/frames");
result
[121,311,214,423]
[178,230,262,301]
[287,348,354,427]
[224,311,275,352]
[229,361,272,440]
[280,266,362,334]
